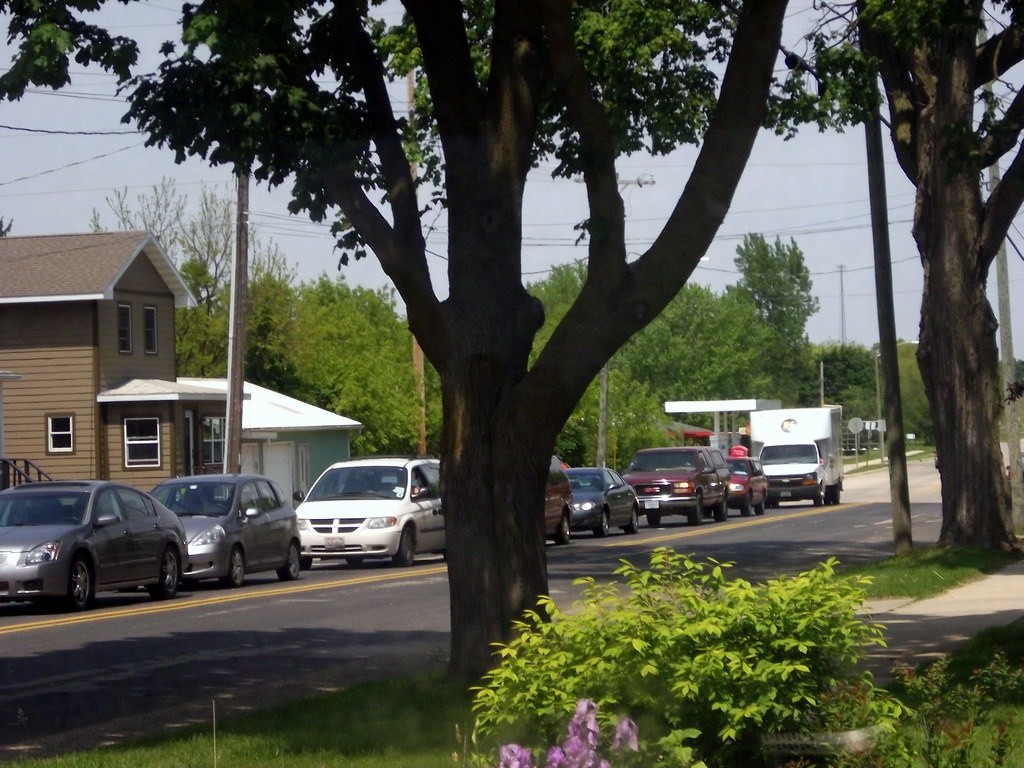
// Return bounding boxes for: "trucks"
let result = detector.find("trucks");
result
[751,405,845,512]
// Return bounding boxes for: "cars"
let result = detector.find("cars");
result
[565,467,640,538]
[294,454,447,571]
[723,445,767,518]
[544,455,573,545]
[149,473,302,590]
[0,479,190,615]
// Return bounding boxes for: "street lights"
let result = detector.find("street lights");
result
[876,340,920,464]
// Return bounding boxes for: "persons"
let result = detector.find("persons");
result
[181,486,226,514]
[393,472,420,496]
[556,455,571,469]
[65,497,87,523]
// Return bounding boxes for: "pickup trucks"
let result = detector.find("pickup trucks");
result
[622,446,730,527]
